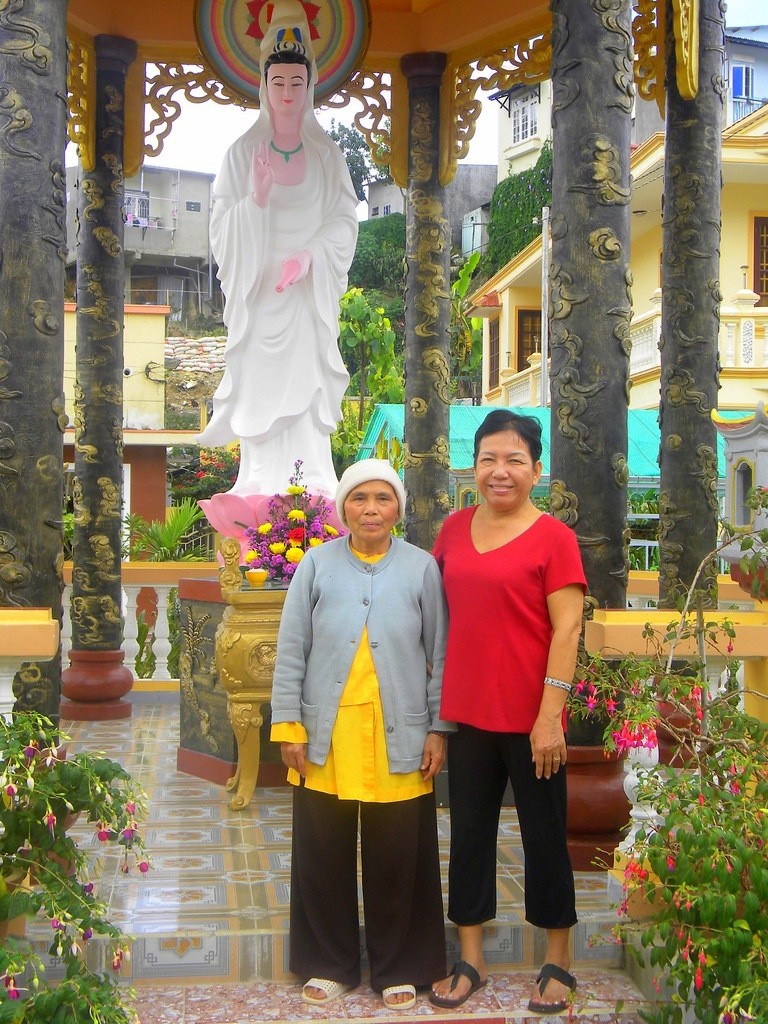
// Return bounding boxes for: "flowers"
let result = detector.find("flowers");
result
[0,713,154,1024]
[246,459,348,586]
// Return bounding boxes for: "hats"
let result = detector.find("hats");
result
[335,459,406,528]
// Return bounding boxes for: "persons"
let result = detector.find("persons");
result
[426,407,590,1016]
[269,459,448,1012]
[190,0,372,577]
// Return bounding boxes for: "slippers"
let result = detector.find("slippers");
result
[429,961,488,1008]
[301,977,353,1005]
[528,963,577,1012]
[382,984,415,1010]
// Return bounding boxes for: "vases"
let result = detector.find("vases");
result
[0,864,28,948]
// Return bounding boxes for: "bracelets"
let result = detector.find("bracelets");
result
[429,731,448,739]
[544,676,573,693]
